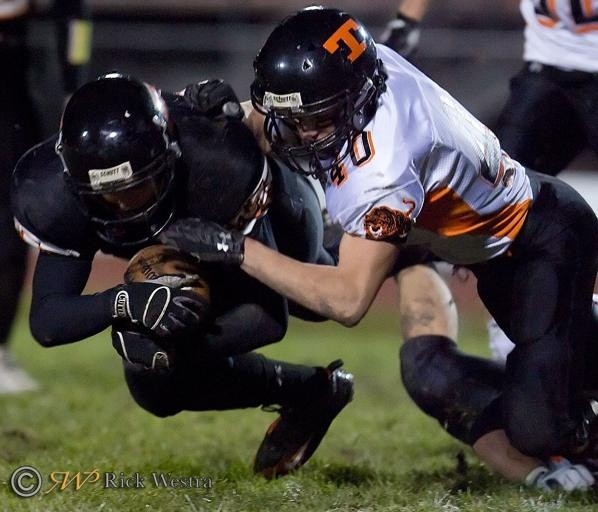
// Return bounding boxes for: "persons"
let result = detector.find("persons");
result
[1,0,58,399]
[152,7,595,491]
[396,261,595,498]
[379,1,596,176]
[8,69,454,482]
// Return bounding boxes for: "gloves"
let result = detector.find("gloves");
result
[111,275,211,375]
[381,12,422,60]
[526,462,597,496]
[184,78,243,121]
[159,215,245,269]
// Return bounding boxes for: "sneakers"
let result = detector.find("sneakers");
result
[253,363,353,479]
[0,348,48,394]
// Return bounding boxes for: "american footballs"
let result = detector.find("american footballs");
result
[124,245,210,315]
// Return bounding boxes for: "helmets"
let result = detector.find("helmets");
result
[54,71,178,249]
[249,4,388,176]
